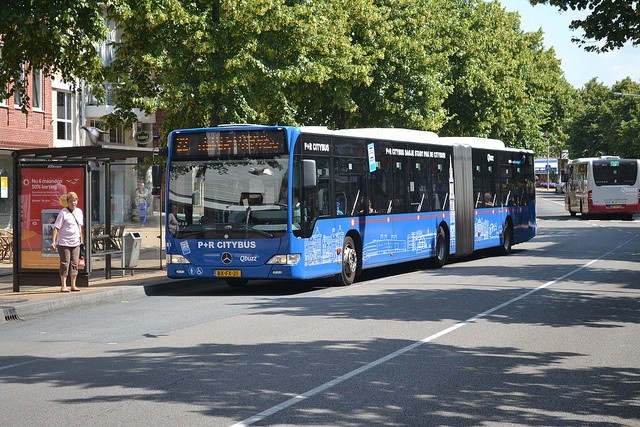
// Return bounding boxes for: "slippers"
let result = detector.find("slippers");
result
[61,287,70,292]
[71,286,80,291]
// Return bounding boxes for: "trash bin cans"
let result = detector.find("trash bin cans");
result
[121,232,142,267]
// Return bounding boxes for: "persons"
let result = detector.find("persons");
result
[359,198,377,213]
[134,181,149,227]
[168,205,181,235]
[51,191,84,291]
[277,182,299,208]
[484,192,493,207]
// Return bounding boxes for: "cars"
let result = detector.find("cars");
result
[540,181,556,188]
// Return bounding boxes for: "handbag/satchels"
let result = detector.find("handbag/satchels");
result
[66,207,85,259]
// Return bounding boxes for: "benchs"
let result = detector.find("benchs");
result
[81,236,125,279]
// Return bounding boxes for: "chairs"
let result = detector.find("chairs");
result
[0,229,14,264]
[91,224,125,252]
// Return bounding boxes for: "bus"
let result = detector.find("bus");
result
[565,156,640,220]
[165,124,536,287]
